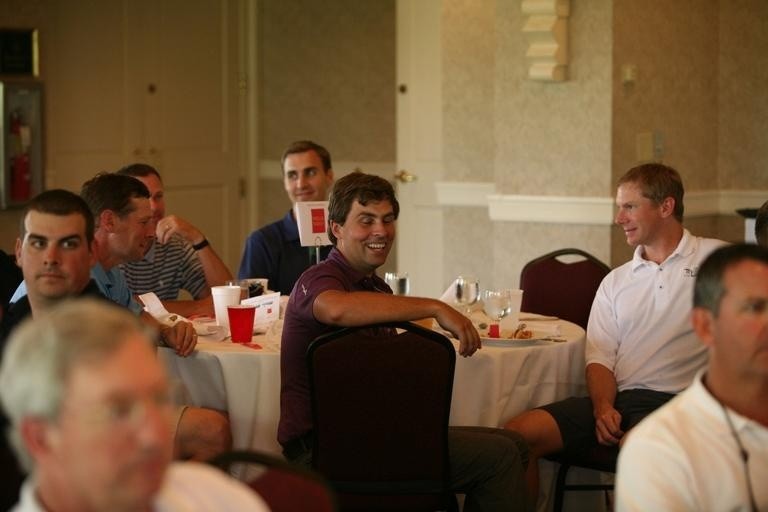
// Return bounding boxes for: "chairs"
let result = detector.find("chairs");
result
[546,440,622,512]
[518,247,612,330]
[302,321,462,512]
[204,447,340,512]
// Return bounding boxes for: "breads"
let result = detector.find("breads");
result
[509,330,532,339]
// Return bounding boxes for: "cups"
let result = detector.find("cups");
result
[229,306,255,343]
[211,281,250,326]
[383,271,410,297]
[500,287,525,336]
[241,278,268,295]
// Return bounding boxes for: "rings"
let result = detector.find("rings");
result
[192,335,196,336]
[166,225,169,229]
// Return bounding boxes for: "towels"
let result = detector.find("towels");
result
[160,313,228,344]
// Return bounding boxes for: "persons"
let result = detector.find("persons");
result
[114,164,235,317]
[0,189,134,512]
[502,164,735,512]
[277,168,530,512]
[10,173,233,462]
[614,241,768,512]
[237,140,337,296]
[0,296,272,512]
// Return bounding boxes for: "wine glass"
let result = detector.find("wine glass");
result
[483,290,511,338]
[453,277,480,321]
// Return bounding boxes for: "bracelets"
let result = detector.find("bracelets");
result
[192,239,210,251]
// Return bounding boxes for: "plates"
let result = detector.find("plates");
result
[472,332,551,344]
